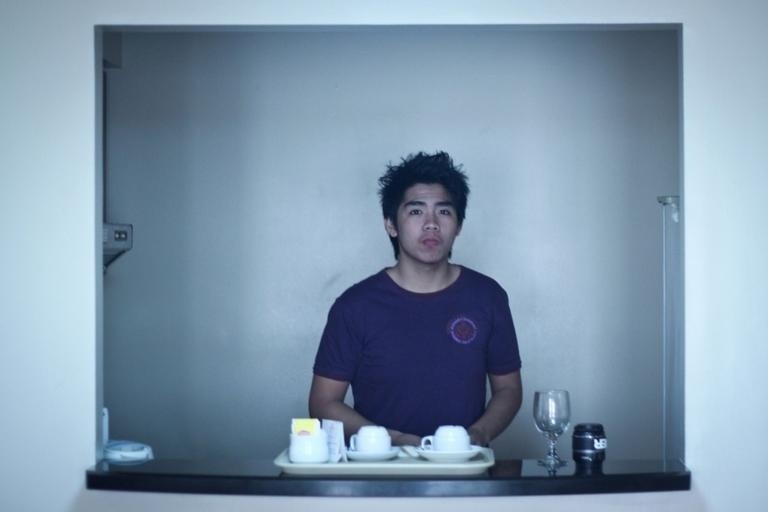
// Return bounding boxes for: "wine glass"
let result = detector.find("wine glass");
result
[532,391,571,466]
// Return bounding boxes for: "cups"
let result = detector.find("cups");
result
[421,425,471,450]
[289,434,330,464]
[350,425,391,450]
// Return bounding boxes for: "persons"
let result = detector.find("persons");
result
[308,149,522,448]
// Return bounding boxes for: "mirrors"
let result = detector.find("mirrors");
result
[86,22,691,497]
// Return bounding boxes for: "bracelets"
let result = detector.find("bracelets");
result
[467,425,490,446]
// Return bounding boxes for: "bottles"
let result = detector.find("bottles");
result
[572,422,608,466]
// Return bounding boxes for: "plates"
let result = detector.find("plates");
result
[416,445,481,461]
[345,446,400,461]
